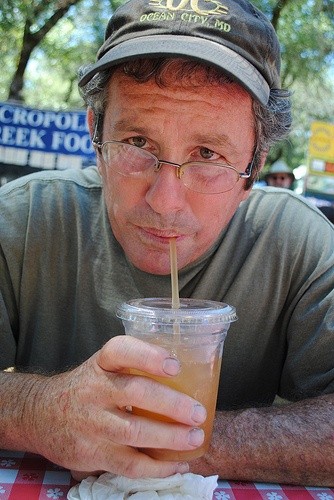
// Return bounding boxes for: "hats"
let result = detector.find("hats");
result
[78,0,280,107]
[265,160,295,184]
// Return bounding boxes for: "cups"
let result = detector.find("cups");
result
[116,297,239,463]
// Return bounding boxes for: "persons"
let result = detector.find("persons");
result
[264,159,295,189]
[0,0,334,487]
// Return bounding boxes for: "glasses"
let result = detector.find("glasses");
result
[92,115,259,194]
[269,176,288,180]
